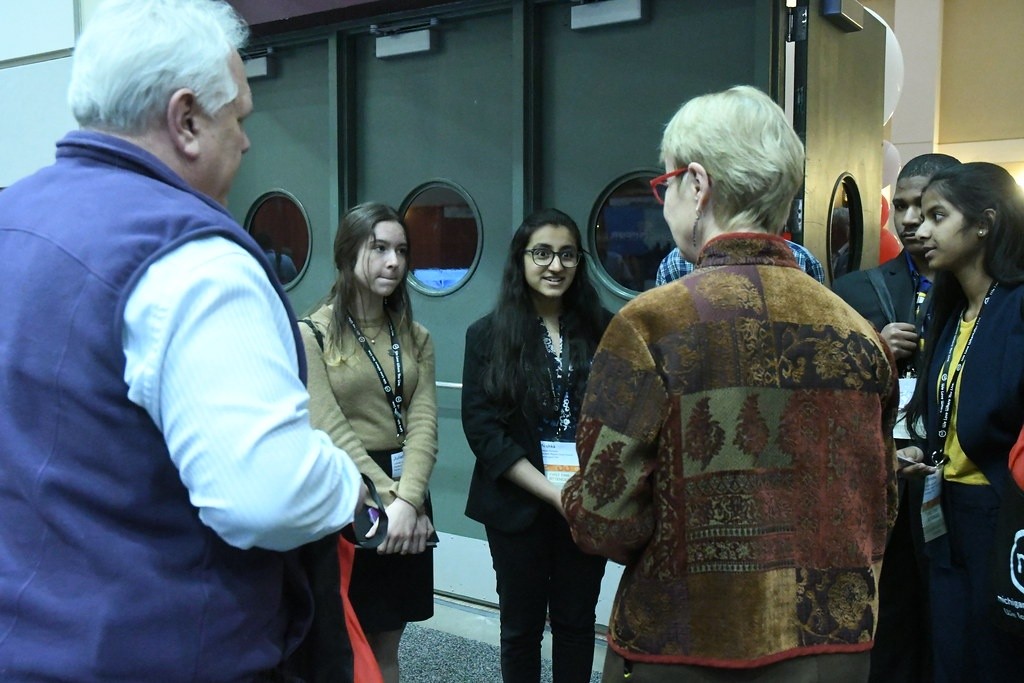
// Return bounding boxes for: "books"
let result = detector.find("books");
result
[355,489,443,548]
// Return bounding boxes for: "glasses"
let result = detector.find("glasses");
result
[525,248,582,268]
[650,167,712,205]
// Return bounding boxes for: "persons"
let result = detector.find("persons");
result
[298,202,437,683]
[829,153,962,682]
[569,87,900,682]
[460,210,615,683]
[897,162,1024,683]
[0,1,366,683]
